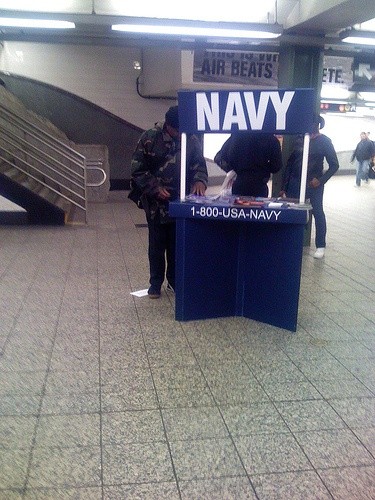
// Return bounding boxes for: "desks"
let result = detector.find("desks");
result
[167,195,314,332]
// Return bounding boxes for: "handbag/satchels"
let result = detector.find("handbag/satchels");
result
[368,162,375,179]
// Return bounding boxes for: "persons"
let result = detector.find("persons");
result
[131,106,208,299]
[281,116,339,259]
[350,131,375,188]
[221,132,282,198]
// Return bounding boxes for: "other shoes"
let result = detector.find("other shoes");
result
[167,284,177,292]
[147,287,161,298]
[315,247,325,258]
